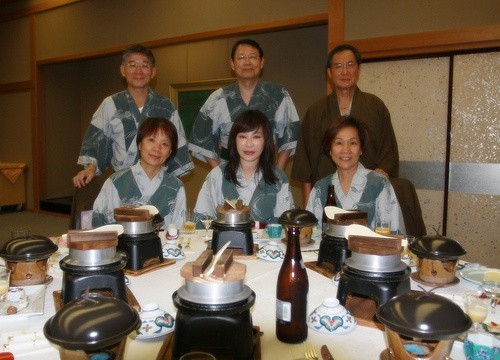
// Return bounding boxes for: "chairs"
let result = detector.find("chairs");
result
[69,170,113,231]
[388,178,427,237]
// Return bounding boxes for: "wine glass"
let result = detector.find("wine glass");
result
[466,302,488,334]
[200,215,214,239]
[480,269,500,332]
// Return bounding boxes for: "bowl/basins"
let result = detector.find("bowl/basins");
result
[306,297,357,335]
[129,303,175,339]
[255,240,285,261]
[161,241,185,259]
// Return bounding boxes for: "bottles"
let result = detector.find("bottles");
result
[321,184,338,233]
[275,227,309,344]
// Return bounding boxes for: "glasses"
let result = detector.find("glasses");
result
[122,62,153,70]
[233,55,261,60]
[329,63,357,69]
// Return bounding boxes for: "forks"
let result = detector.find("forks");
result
[302,340,318,360]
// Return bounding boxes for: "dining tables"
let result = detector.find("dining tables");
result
[0,227,500,360]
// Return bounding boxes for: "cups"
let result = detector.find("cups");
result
[374,219,391,235]
[0,267,12,301]
[266,224,282,237]
[400,234,415,265]
[183,210,196,233]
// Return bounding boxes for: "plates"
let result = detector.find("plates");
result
[459,266,500,290]
[0,284,46,317]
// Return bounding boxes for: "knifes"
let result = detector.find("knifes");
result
[320,345,334,360]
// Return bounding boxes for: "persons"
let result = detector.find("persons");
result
[289,44,398,208]
[92,117,187,229]
[188,39,300,171]
[195,109,295,229]
[306,116,407,237]
[73,46,194,187]
